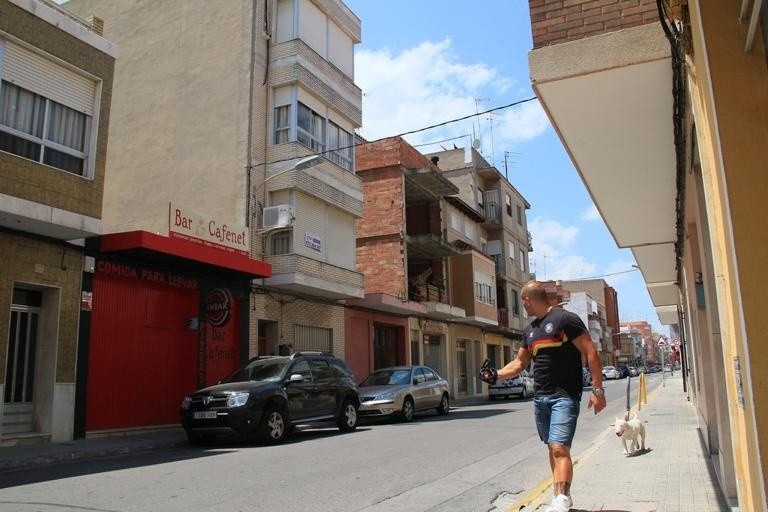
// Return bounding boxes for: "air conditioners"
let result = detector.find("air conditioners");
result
[262,204,293,233]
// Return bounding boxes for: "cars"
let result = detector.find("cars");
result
[583,365,661,387]
[487,370,534,400]
[664,364,673,372]
[180,351,450,446]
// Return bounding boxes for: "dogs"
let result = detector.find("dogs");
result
[614,411,646,457]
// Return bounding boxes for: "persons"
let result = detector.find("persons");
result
[476,281,608,511]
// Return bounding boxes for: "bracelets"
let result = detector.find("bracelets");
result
[591,387,604,396]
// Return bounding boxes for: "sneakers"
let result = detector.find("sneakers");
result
[544,491,574,512]
